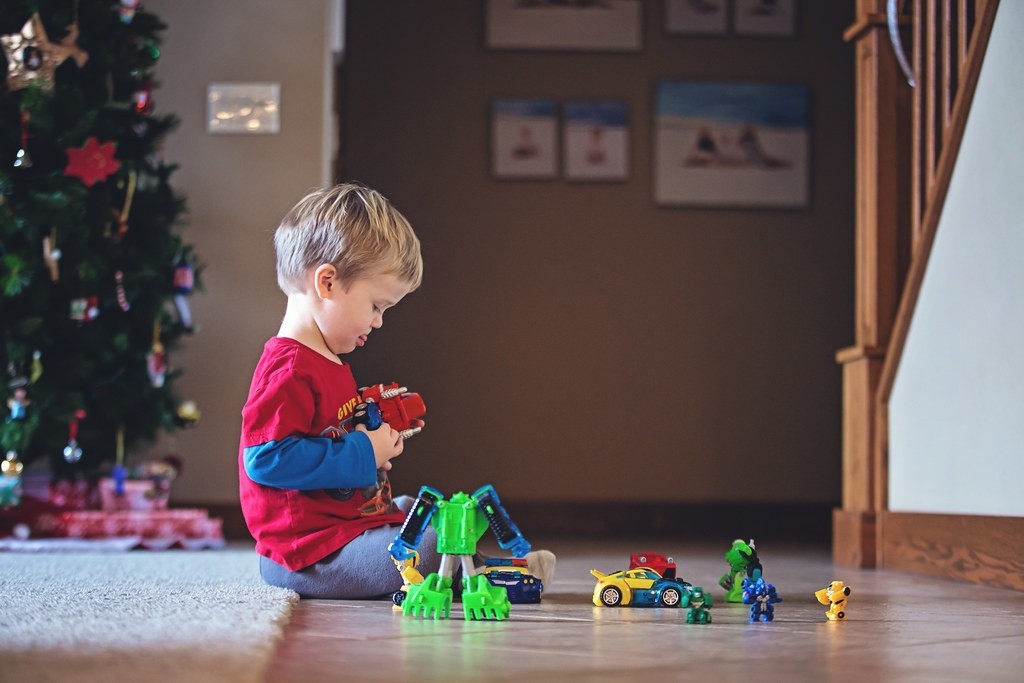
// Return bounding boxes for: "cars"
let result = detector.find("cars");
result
[590,568,693,611]
[485,556,546,604]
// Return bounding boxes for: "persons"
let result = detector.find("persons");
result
[235,181,556,600]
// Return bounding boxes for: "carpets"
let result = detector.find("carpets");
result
[1,543,304,682]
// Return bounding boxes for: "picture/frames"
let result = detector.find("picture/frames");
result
[648,76,815,210]
[555,99,633,179]
[660,1,733,38]
[488,96,564,181]
[729,0,801,39]
[483,0,645,56]
